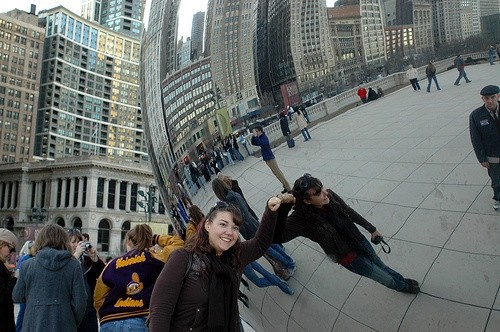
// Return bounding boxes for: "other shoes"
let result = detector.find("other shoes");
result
[406,279,418,286]
[493,201,500,209]
[286,288,294,295]
[406,286,420,294]
[282,189,287,193]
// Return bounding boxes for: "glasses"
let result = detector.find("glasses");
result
[7,245,14,253]
[65,229,80,234]
[300,173,312,187]
[209,202,237,216]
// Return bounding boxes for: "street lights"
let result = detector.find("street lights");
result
[137,201,148,224]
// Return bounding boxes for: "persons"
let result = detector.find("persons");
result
[93,223,185,332]
[64,226,106,332]
[406,64,421,92]
[357,85,384,104]
[279,104,311,148]
[251,125,291,194]
[105,256,112,265]
[496,42,500,59]
[454,53,472,85]
[272,172,421,294]
[15,241,33,332]
[488,44,496,65]
[469,85,500,210]
[150,196,282,332]
[184,134,252,196]
[426,62,441,92]
[12,223,88,332]
[170,172,295,308]
[0,227,18,332]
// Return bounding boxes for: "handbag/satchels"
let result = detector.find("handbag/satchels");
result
[287,139,296,148]
[262,252,290,281]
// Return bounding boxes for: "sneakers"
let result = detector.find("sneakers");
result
[291,259,296,264]
[288,265,296,277]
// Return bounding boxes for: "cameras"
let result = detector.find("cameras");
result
[371,235,383,246]
[82,242,91,250]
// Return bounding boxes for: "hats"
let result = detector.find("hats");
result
[0,228,18,247]
[480,85,500,95]
